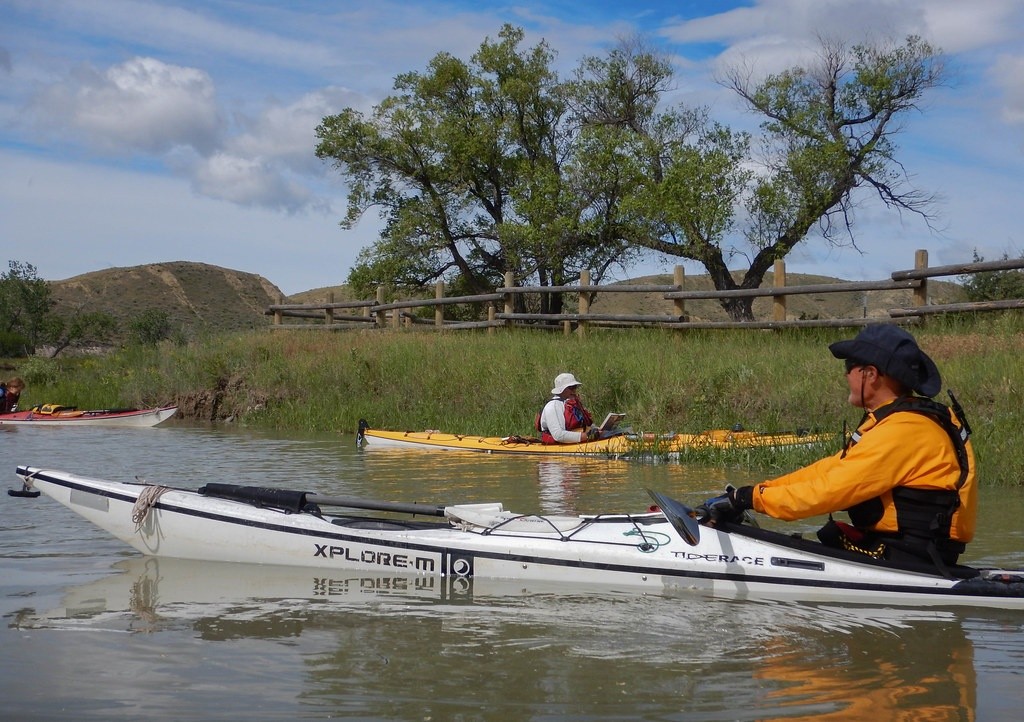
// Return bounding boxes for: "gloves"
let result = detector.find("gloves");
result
[703,486,754,524]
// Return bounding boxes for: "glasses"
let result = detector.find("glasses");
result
[845,360,884,376]
[567,385,577,390]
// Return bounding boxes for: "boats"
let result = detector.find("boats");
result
[16,464,1024,608]
[15,556,952,668]
[359,421,847,459]
[0,404,179,427]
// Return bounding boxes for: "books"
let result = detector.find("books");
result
[600,412,625,433]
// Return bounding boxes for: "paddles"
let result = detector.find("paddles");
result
[122,481,585,533]
[51,402,170,418]
[642,485,760,547]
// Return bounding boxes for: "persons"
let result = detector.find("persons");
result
[540,373,601,443]
[0,377,26,415]
[698,323,979,575]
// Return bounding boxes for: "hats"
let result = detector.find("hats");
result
[828,323,942,398]
[551,373,582,394]
